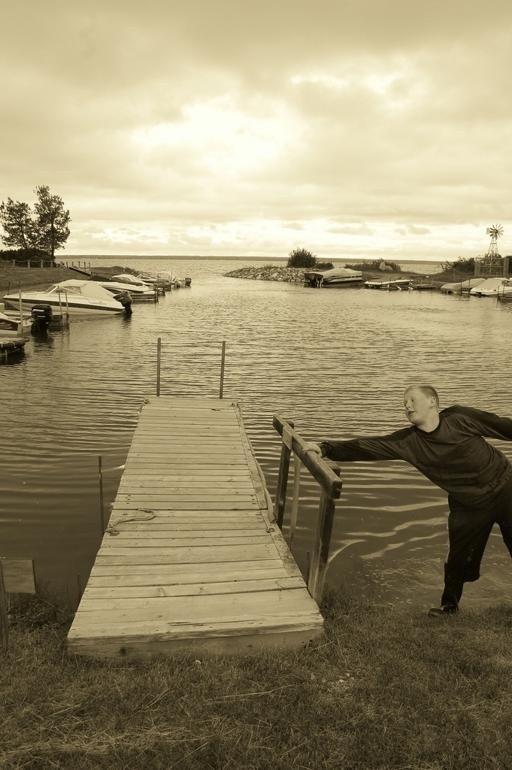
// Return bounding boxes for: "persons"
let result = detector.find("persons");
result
[301,382,511,621]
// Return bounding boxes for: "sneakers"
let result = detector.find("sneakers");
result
[428,603,459,617]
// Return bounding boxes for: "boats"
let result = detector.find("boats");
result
[303,266,512,303]
[1,270,196,360]
